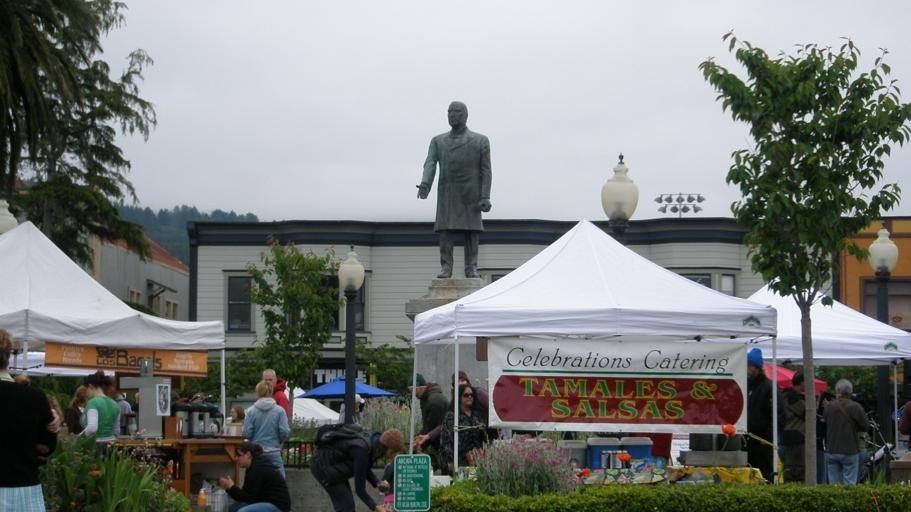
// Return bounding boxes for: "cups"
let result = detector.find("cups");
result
[140,358,154,377]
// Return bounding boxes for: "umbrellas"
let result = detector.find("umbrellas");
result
[295,374,396,400]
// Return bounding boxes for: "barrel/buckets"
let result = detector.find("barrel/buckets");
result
[203,487,229,512]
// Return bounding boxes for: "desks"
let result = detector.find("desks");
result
[664,463,766,485]
[115,437,245,497]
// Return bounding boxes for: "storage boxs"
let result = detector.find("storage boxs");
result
[559,438,589,465]
[620,436,655,468]
[586,437,622,468]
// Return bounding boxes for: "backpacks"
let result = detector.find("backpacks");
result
[311,421,372,458]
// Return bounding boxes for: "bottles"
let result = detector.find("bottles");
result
[378,487,385,507]
[215,413,243,436]
[198,480,230,511]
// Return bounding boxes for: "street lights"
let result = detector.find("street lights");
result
[337,244,365,426]
[651,190,707,221]
[599,153,639,243]
[0,200,18,235]
[864,228,898,444]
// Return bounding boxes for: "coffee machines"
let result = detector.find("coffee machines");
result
[118,375,173,439]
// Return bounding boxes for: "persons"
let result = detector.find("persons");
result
[14,373,60,436]
[336,393,365,425]
[241,379,291,482]
[64,372,139,439]
[416,100,494,280]
[746,347,871,485]
[0,326,57,512]
[261,368,292,430]
[216,440,291,512]
[221,407,245,436]
[307,422,404,512]
[405,370,501,475]
[381,456,396,512]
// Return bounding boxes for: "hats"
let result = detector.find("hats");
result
[407,372,426,388]
[379,429,407,452]
[747,347,763,368]
[355,393,365,404]
